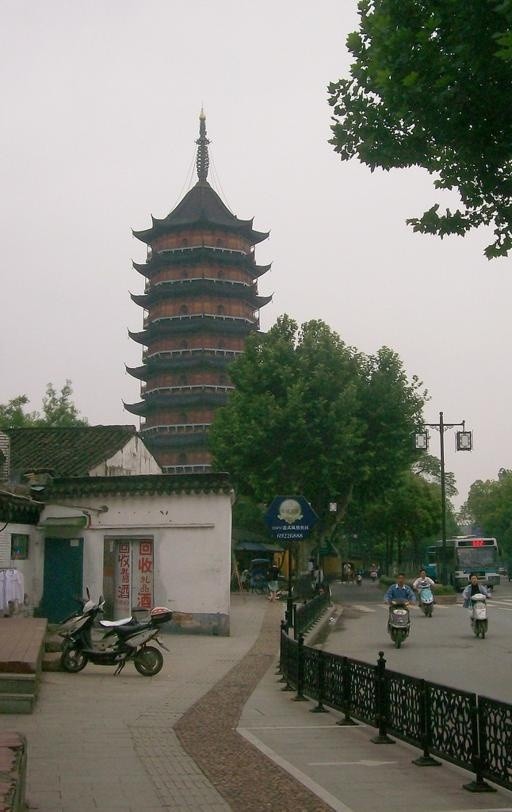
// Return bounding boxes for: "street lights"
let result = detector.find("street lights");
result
[414,412,471,592]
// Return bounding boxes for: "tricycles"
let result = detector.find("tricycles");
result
[341,562,355,584]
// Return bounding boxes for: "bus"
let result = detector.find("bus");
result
[425,535,501,593]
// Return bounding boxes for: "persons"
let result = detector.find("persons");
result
[343,561,378,584]
[462,575,491,608]
[266,561,279,602]
[384,574,416,609]
[413,571,434,589]
[309,558,315,574]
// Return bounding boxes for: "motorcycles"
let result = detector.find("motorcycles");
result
[417,580,434,617]
[356,575,362,584]
[59,585,172,676]
[384,599,416,649]
[369,569,378,582]
[470,594,488,639]
